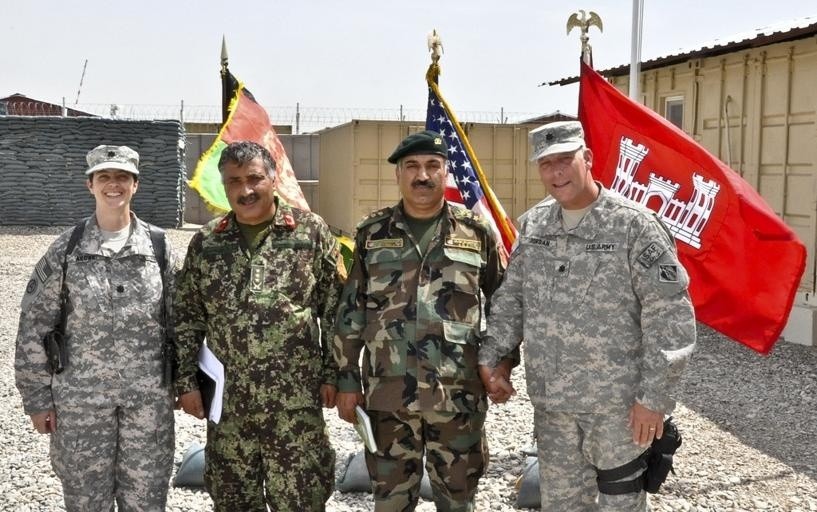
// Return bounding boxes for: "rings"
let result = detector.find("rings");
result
[649,427,655,430]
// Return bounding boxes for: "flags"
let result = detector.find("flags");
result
[423,28,519,277]
[182,34,354,278]
[562,6,810,358]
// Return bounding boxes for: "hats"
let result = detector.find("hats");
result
[84,144,140,176]
[528,120,587,162]
[387,129,449,164]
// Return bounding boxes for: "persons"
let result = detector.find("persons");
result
[15,140,183,512]
[476,119,699,512]
[169,138,350,511]
[327,127,524,512]
